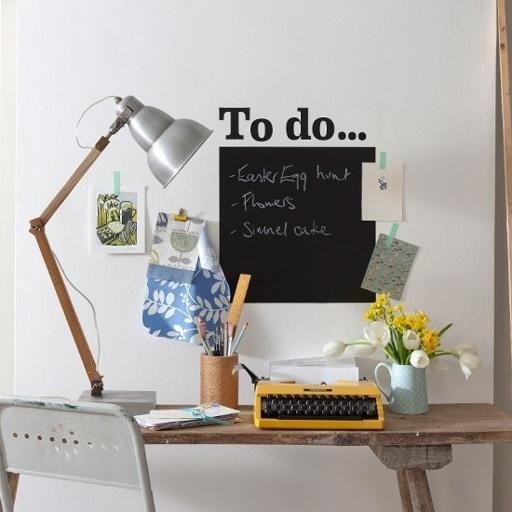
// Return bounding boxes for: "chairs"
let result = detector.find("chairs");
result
[0,399,155,512]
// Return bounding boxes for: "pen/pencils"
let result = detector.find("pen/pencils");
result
[197,315,249,357]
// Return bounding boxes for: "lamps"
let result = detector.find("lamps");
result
[25,90,216,418]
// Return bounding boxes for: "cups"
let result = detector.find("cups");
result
[198,350,239,411]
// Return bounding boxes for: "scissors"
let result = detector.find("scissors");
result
[239,362,268,394]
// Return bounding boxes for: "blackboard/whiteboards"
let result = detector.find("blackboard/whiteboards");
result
[219,146,375,303]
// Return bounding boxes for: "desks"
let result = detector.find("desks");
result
[0,399,510,511]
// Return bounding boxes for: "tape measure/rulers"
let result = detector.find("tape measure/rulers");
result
[226,273,252,324]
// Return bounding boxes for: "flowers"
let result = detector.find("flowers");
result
[322,288,482,380]
